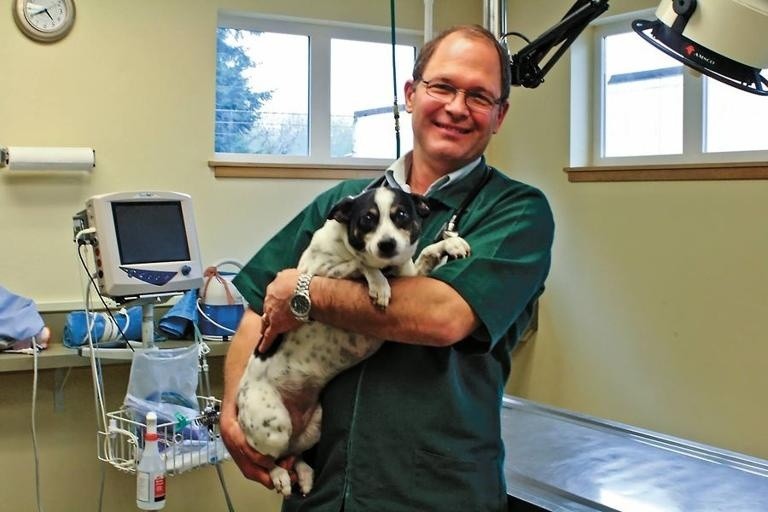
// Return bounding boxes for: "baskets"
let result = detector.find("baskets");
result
[104,393,231,477]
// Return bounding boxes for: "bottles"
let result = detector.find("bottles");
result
[133,413,168,512]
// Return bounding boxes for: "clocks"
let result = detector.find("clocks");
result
[12,1,79,46]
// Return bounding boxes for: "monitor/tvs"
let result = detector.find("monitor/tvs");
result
[85,191,204,299]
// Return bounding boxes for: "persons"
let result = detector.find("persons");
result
[216,23,554,512]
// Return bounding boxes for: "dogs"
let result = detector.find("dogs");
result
[233,185,472,498]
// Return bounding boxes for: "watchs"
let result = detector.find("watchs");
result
[289,273,313,324]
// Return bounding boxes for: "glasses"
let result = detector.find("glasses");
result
[418,75,504,114]
